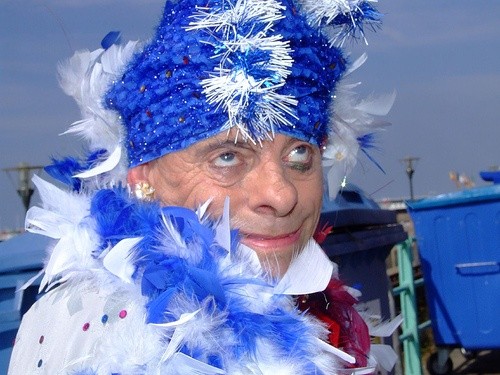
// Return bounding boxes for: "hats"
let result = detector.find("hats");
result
[100,1,346,170]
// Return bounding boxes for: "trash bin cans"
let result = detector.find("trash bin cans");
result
[0,232,62,374]
[309,181,409,375]
[401,182,500,355]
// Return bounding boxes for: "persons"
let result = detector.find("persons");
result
[6,0,407,375]
